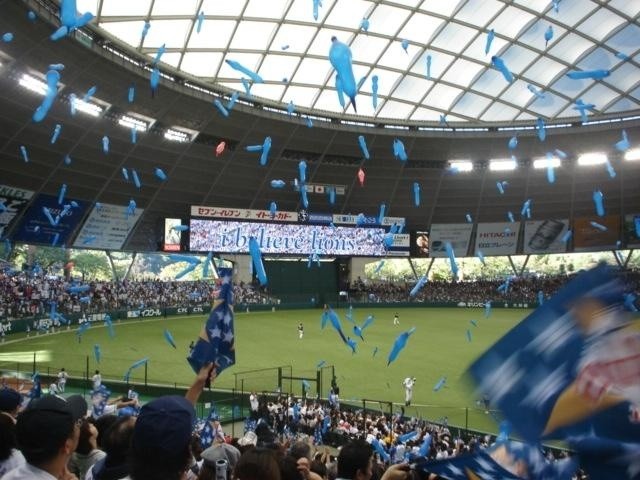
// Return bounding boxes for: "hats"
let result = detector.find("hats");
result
[0,388,24,408]
[134,396,195,456]
[200,443,241,472]
[15,395,87,446]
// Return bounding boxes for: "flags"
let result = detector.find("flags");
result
[185,266,236,389]
[460,260,639,479]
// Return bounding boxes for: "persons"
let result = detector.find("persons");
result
[296,323,304,339]
[1,365,587,479]
[391,311,401,328]
[0,254,281,343]
[351,272,640,318]
